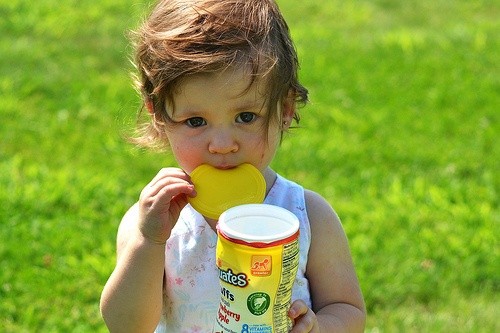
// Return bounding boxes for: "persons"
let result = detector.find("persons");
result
[98,0,368,333]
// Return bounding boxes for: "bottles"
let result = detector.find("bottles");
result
[212,203,301,333]
